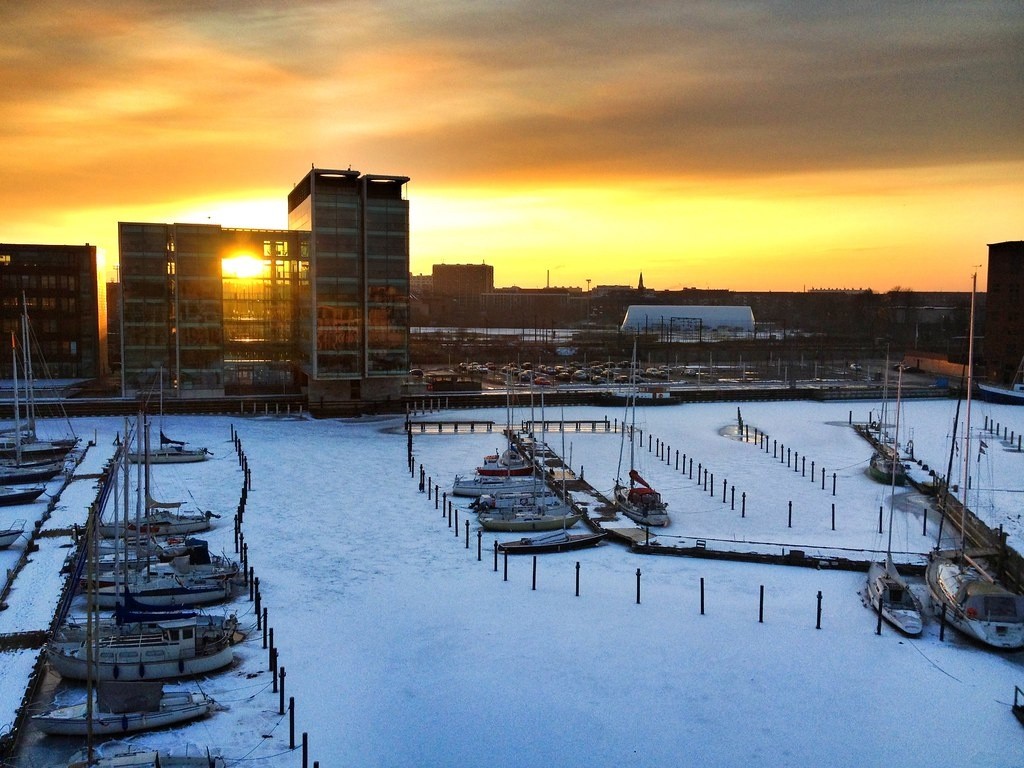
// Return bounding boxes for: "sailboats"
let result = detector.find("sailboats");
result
[497,406,608,555]
[923,272,1024,651]
[0,289,83,507]
[126,366,214,464]
[452,362,585,534]
[612,334,668,527]
[864,366,924,638]
[868,343,905,486]
[29,409,229,767]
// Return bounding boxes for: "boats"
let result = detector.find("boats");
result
[977,354,1024,406]
[0,518,28,551]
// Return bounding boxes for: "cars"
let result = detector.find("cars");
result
[409,369,423,378]
[849,363,862,371]
[457,358,696,384]
